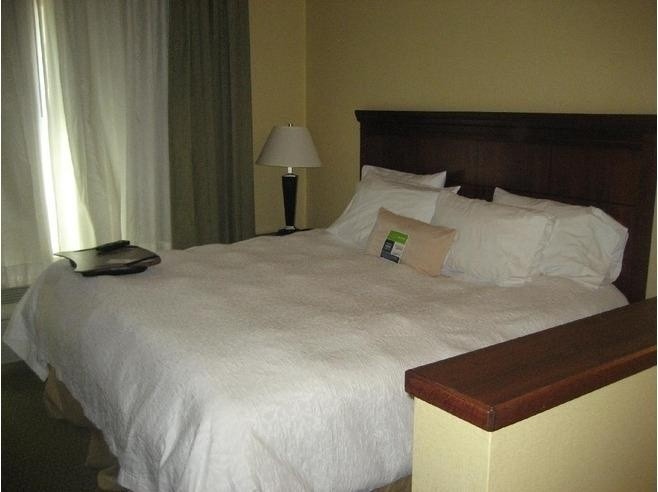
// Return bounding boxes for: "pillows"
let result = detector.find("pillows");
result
[366,206,457,278]
[326,172,461,255]
[362,164,446,191]
[493,186,631,288]
[430,189,555,287]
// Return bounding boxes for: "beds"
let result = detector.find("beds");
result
[2,110,657,492]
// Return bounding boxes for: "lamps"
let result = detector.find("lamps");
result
[255,121,324,233]
